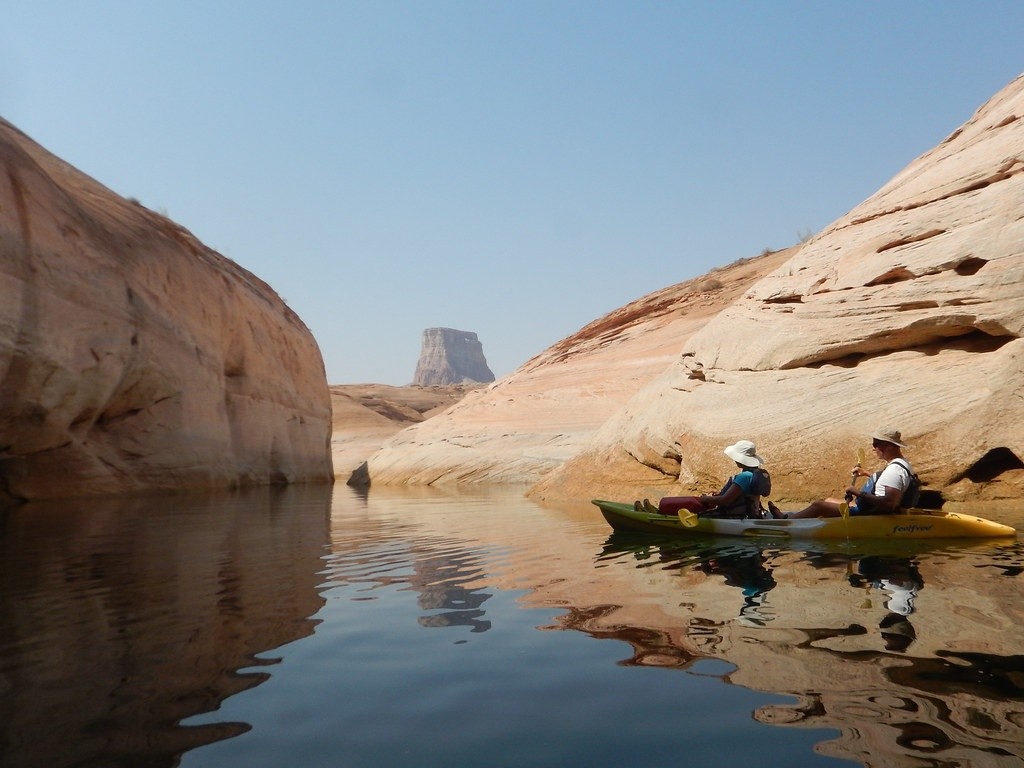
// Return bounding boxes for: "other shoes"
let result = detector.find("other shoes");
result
[767,501,786,519]
[634,501,645,512]
[643,499,658,513]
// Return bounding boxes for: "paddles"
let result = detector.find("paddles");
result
[675,506,721,527]
[839,447,866,520]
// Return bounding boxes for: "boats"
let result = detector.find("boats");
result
[590,499,1017,542]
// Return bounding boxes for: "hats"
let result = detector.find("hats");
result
[724,440,764,467]
[868,424,907,448]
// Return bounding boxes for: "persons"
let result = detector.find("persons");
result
[634,441,771,519]
[768,431,921,518]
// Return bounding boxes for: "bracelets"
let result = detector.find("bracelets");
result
[859,491,867,497]
